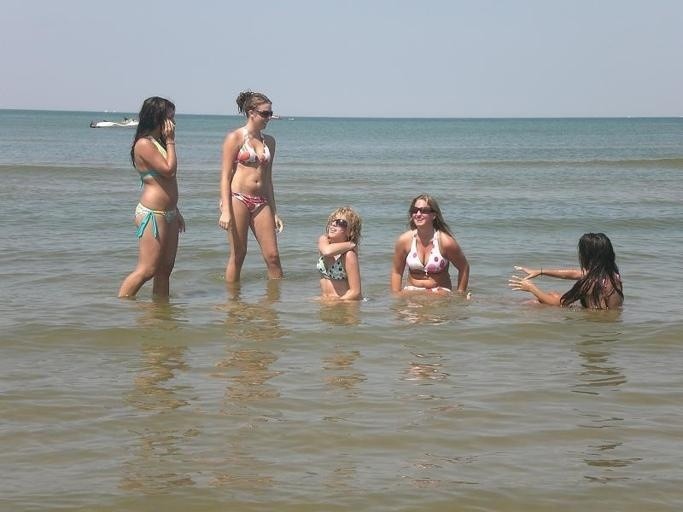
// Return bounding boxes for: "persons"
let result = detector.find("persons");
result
[316,206,363,304]
[390,195,469,305]
[117,96,186,299]
[218,92,284,283]
[507,232,624,311]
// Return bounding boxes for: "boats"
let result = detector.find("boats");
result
[89,119,140,128]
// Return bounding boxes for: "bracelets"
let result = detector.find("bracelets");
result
[166,140,175,145]
[540,267,542,275]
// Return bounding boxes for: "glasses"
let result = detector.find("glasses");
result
[412,206,431,214]
[329,218,347,227]
[254,108,272,118]
